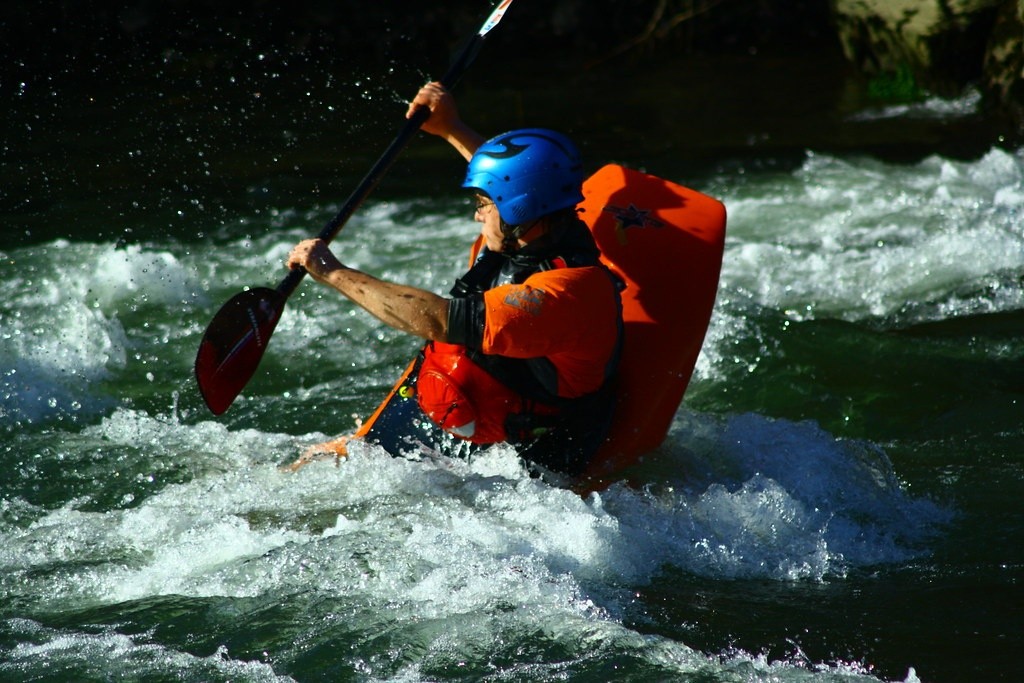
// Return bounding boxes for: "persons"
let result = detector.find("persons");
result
[286,81,624,457]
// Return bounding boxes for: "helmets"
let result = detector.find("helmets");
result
[461,128,586,227]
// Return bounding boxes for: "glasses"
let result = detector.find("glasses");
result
[475,195,495,214]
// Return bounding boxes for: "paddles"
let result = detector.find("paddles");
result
[197,0,509,411]
